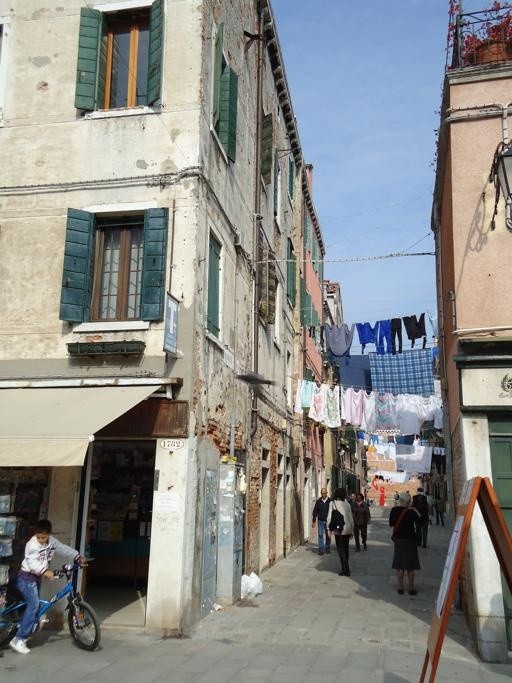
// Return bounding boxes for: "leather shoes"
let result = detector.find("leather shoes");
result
[338,569,352,576]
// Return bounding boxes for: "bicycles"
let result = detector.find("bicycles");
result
[1,557,101,650]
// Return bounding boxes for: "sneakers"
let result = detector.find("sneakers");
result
[397,587,418,595]
[8,636,31,655]
[318,547,331,555]
[356,544,368,552]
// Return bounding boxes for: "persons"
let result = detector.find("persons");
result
[389,486,444,547]
[9,518,89,653]
[388,492,426,595]
[305,486,371,576]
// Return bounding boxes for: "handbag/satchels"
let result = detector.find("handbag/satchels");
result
[329,501,346,533]
[391,525,400,542]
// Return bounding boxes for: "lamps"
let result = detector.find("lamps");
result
[490,137,512,232]
